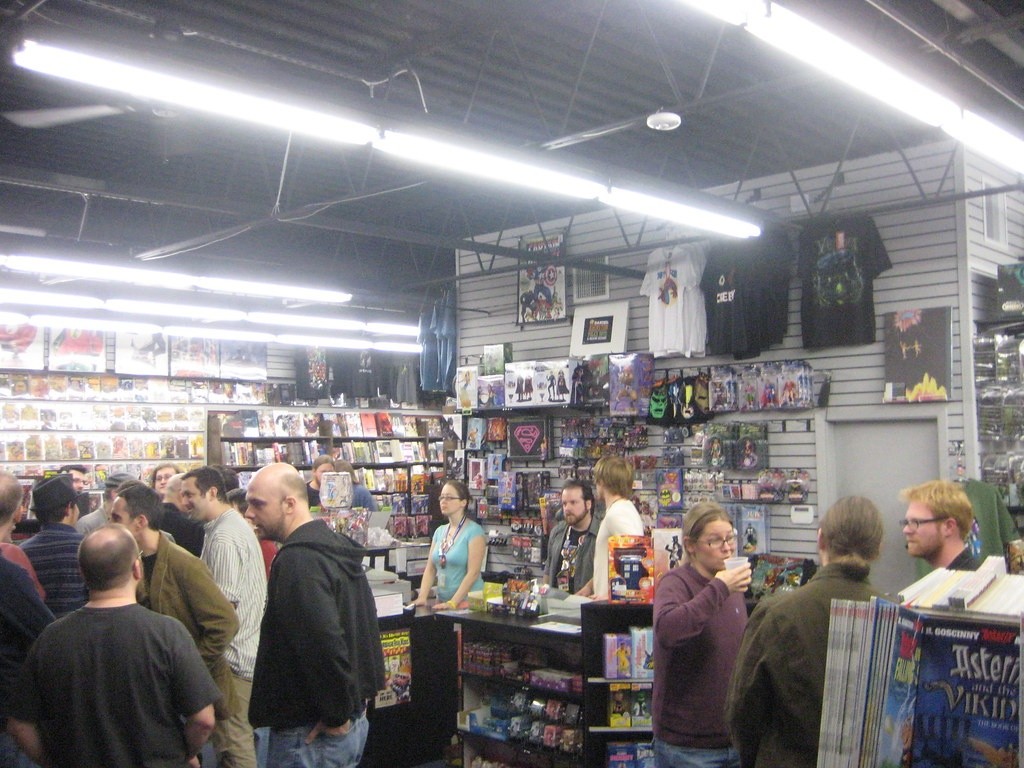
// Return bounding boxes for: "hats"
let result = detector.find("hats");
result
[105,474,135,486]
[30,474,89,511]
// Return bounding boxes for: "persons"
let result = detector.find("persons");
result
[543,479,601,599]
[243,464,387,768]
[576,455,645,601]
[651,500,752,768]
[403,480,488,613]
[898,482,983,573]
[724,497,887,768]
[303,455,376,513]
[0,464,278,768]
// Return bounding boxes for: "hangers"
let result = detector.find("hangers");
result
[645,219,699,259]
[422,280,456,305]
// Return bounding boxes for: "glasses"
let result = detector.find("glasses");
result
[694,534,736,548]
[438,496,467,501]
[900,517,948,530]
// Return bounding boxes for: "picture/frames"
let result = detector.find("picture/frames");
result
[570,300,630,357]
[517,227,568,325]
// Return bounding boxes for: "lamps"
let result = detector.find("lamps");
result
[1,250,422,360]
[10,1,1024,243]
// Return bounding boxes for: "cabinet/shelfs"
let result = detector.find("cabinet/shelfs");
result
[1,372,267,503]
[580,603,658,768]
[440,614,581,768]
[211,412,457,556]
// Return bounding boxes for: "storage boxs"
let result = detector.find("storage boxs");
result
[483,343,513,376]
[606,353,656,419]
[456,366,479,410]
[505,357,585,405]
[479,375,505,408]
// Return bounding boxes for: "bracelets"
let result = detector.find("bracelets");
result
[446,601,457,612]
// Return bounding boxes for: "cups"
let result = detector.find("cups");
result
[723,557,748,575]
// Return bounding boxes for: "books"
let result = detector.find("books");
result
[817,597,1024,768]
